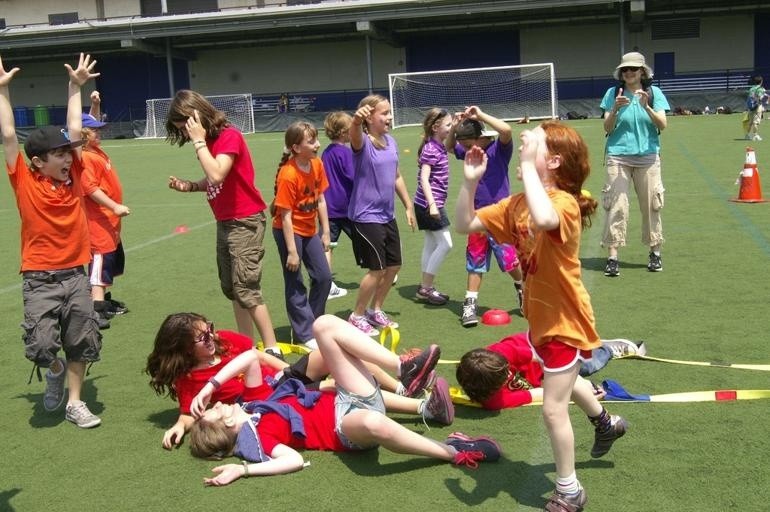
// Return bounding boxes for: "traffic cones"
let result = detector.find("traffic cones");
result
[727,149,769,202]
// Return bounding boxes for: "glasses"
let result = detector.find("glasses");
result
[621,67,641,72]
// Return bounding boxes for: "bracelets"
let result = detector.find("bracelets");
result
[187,180,193,192]
[194,183,199,191]
[196,145,207,151]
[193,141,207,146]
[612,109,618,114]
[352,121,363,126]
[208,376,220,389]
[429,201,434,208]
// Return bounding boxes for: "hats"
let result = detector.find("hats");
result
[64,113,109,130]
[455,118,499,138]
[613,52,653,81]
[24,125,86,159]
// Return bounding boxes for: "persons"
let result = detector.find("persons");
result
[270,122,332,349]
[456,334,640,411]
[319,112,398,300]
[348,95,415,337]
[745,77,770,141]
[165,89,284,360]
[0,52,103,428]
[141,312,455,449]
[279,94,288,113]
[190,314,501,486]
[455,121,626,512]
[66,91,129,315]
[446,106,525,326]
[600,52,670,276]
[414,108,452,305]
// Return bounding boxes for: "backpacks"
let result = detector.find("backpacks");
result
[747,87,761,111]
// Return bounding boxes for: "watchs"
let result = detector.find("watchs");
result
[242,461,249,478]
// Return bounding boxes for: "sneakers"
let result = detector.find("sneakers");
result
[106,304,128,315]
[349,313,379,335]
[400,344,440,398]
[601,339,639,359]
[327,282,348,300]
[64,401,100,428]
[44,358,69,411]
[544,483,586,511]
[415,288,448,306]
[426,378,452,422]
[462,304,478,326]
[365,310,399,329]
[648,252,663,272]
[446,432,500,467]
[93,312,110,329]
[604,258,620,277]
[591,416,626,458]
